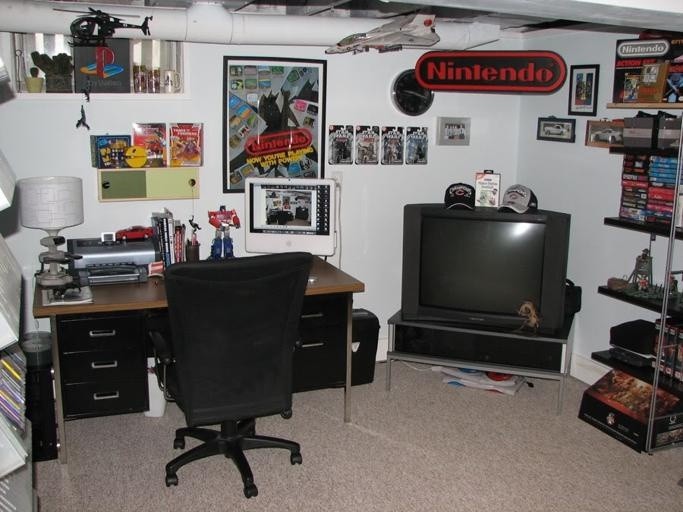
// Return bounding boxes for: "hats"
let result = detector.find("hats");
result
[444,183,475,211]
[497,184,537,214]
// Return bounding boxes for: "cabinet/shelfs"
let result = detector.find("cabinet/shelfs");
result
[289,294,347,397]
[588,101,683,403]
[54,311,151,420]
[384,310,572,416]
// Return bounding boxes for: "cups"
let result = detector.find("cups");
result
[132,65,147,92]
[186,241,200,261]
[165,70,183,93]
[148,67,161,93]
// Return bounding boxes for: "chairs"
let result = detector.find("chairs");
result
[144,252,315,497]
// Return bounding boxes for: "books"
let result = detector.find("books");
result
[40,286,94,307]
[650,317,683,385]
[0,341,28,479]
[150,209,186,267]
[618,153,682,226]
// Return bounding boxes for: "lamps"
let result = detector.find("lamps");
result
[14,175,87,286]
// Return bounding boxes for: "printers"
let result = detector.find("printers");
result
[65,235,162,289]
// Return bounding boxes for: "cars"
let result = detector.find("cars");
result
[591,129,621,144]
[544,124,564,135]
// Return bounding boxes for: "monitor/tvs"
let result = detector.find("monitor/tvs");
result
[244,177,337,257]
[401,201,572,336]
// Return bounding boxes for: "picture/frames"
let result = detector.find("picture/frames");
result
[535,116,578,144]
[565,61,601,117]
[435,117,471,147]
[220,53,329,196]
[584,118,625,148]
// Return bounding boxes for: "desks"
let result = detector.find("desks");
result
[29,254,367,464]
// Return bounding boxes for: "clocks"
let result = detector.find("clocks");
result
[392,68,435,117]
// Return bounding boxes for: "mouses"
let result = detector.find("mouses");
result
[309,274,318,284]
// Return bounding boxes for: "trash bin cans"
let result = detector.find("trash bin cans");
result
[144,356,167,417]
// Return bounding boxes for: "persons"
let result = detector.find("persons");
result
[207,203,242,261]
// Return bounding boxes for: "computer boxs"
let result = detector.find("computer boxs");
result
[350,307,380,387]
